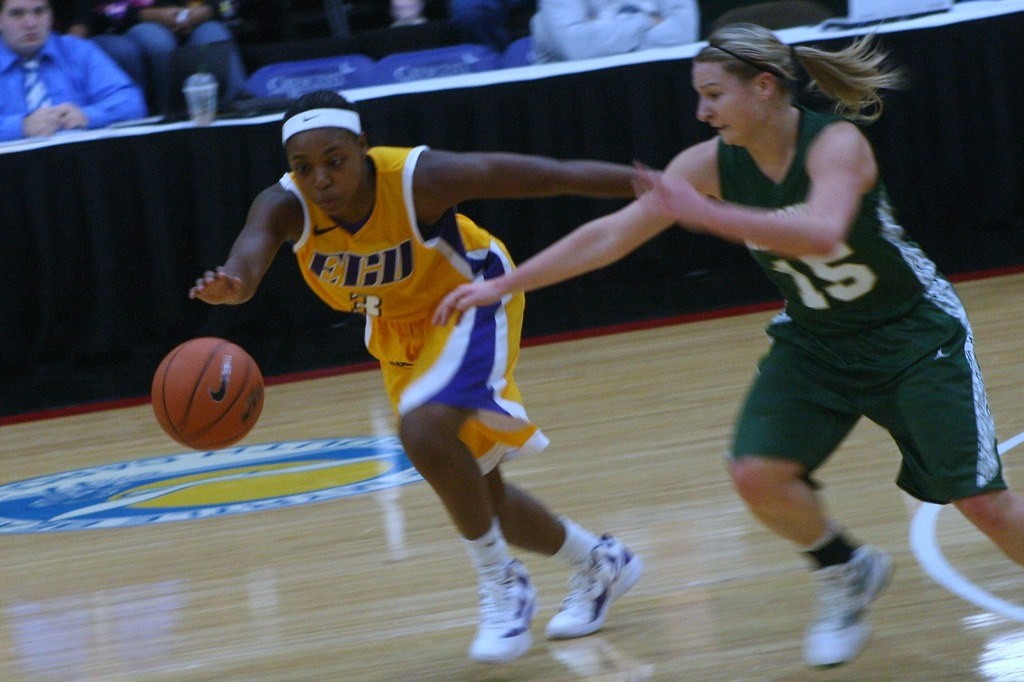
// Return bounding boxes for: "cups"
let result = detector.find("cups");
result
[182,72,219,123]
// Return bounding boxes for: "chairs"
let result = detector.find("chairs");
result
[80,35,542,116]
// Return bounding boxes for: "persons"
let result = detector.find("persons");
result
[188,85,659,666]
[525,0,700,70]
[55,0,526,116]
[435,24,1024,672]
[0,0,148,143]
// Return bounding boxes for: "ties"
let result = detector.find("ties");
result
[24,60,52,115]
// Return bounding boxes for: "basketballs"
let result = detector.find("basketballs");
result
[149,336,267,451]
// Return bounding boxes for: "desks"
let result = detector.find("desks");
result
[0,0,1024,415]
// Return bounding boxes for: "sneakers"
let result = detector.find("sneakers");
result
[804,544,895,666]
[546,533,645,638]
[470,559,539,664]
